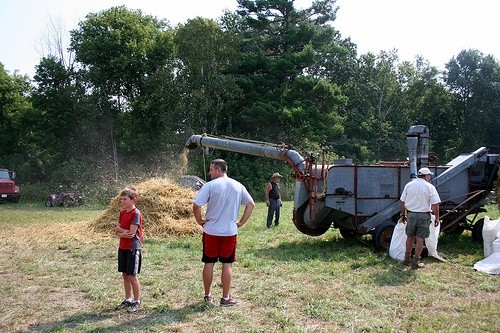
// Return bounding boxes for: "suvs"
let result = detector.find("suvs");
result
[0,168,21,203]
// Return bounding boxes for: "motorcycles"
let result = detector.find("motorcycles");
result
[44,185,84,208]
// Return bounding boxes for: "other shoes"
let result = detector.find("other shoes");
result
[219,294,241,307]
[204,295,214,303]
[115,299,133,310]
[126,300,143,313]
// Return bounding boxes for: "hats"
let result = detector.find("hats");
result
[418,167,435,175]
[271,172,283,178]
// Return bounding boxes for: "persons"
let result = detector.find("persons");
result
[115,191,143,312]
[388,211,429,262]
[265,173,283,228]
[193,159,255,307]
[399,167,441,269]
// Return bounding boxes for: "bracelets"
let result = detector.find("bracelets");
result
[400,214,405,217]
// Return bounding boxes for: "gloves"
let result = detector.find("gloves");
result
[279,200,283,207]
[266,201,270,207]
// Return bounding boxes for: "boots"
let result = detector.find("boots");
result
[402,252,411,266]
[411,255,425,270]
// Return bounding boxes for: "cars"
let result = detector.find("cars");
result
[176,173,205,191]
